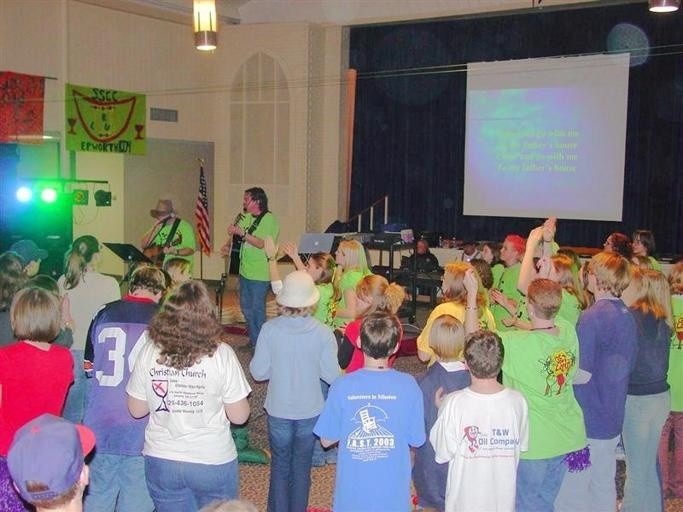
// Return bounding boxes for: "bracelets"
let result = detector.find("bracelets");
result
[267,256,276,261]
[466,306,478,310]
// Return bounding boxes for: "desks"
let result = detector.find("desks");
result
[371,266,445,310]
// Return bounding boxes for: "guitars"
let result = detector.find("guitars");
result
[225,212,247,276]
[144,238,181,268]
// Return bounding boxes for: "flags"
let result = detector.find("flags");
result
[64,82,148,157]
[0,70,46,142]
[195,166,213,257]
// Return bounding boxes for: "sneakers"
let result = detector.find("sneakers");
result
[235,342,253,353]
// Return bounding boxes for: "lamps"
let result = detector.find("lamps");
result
[648,1,679,12]
[40,188,58,206]
[193,0,217,51]
[95,189,112,208]
[72,190,89,207]
[17,185,31,201]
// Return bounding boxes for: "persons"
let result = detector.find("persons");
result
[429,328,529,512]
[312,312,427,512]
[83,267,167,512]
[5,414,96,512]
[332,240,374,333]
[406,239,440,271]
[56,234,121,351]
[516,225,582,329]
[125,280,252,512]
[220,187,281,354]
[249,270,345,512]
[480,241,497,268]
[462,268,589,511]
[487,234,525,332]
[491,289,531,329]
[554,250,639,512]
[417,262,496,371]
[141,199,196,284]
[454,236,481,263]
[0,286,75,512]
[10,238,49,278]
[631,255,654,271]
[164,258,192,292]
[631,229,659,270]
[334,274,406,374]
[539,215,578,278]
[619,268,675,511]
[410,314,472,512]
[262,235,336,402]
[0,250,73,348]
[658,260,683,511]
[602,232,633,262]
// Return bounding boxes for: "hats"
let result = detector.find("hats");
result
[9,239,48,266]
[458,236,480,246]
[276,270,320,308]
[7,412,96,500]
[150,199,175,216]
[73,234,103,259]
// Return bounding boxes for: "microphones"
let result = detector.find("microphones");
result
[243,198,253,211]
[157,212,175,225]
